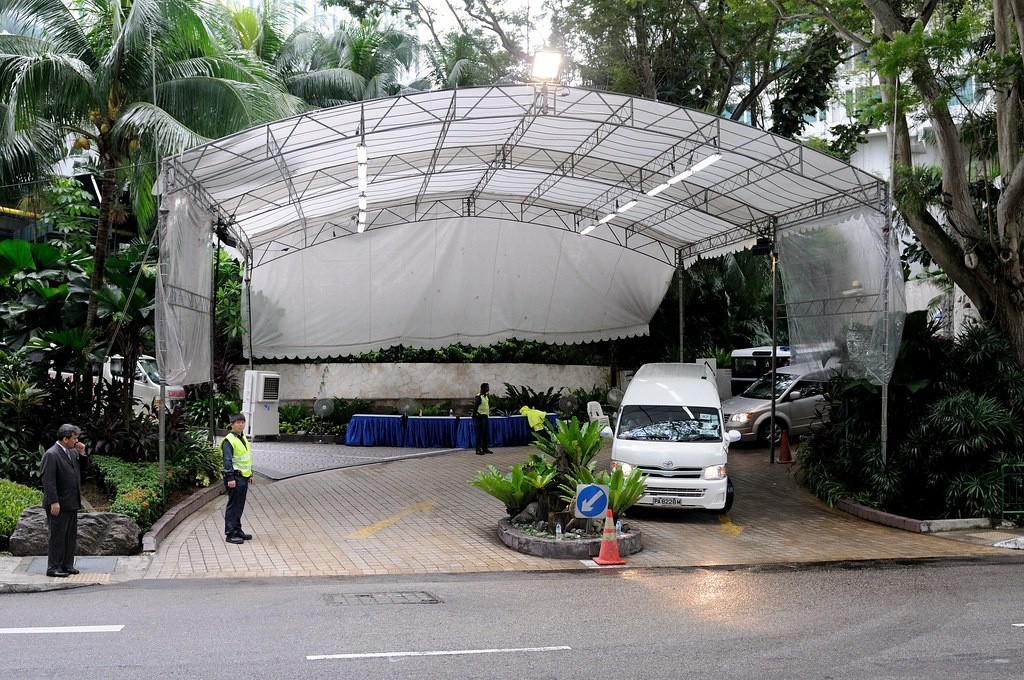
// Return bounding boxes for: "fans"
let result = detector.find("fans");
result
[397,396,417,418]
[607,389,625,408]
[313,397,334,444]
[559,393,577,429]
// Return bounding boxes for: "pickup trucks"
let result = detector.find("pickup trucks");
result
[47,354,187,426]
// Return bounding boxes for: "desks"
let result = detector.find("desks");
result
[345,413,559,448]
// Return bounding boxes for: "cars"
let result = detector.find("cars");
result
[720,359,868,449]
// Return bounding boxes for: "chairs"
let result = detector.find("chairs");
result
[587,400,610,427]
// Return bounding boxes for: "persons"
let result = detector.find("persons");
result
[219,413,255,545]
[781,358,789,367]
[40,424,86,578]
[471,383,494,455]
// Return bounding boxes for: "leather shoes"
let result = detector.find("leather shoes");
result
[58,568,79,574]
[226,534,244,544]
[47,569,70,577]
[476,450,484,455]
[234,531,252,539]
[482,450,493,453]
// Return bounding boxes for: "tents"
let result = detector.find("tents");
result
[150,81,910,474]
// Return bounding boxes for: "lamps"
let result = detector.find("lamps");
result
[351,103,369,233]
[575,116,723,235]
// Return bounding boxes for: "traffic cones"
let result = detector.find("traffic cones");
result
[593,508,626,566]
[775,429,798,464]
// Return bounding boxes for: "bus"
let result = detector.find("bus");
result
[730,342,866,398]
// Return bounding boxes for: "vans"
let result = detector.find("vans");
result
[601,362,741,515]
